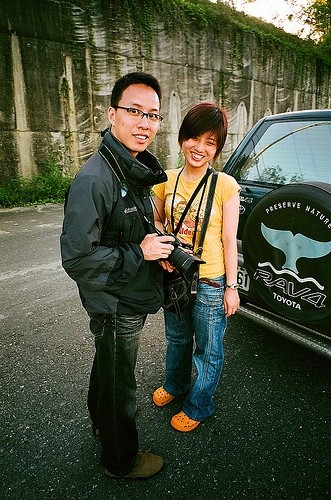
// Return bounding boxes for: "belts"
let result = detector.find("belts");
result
[199,278,222,289]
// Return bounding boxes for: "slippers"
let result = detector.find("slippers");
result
[170,410,201,432]
[152,385,174,407]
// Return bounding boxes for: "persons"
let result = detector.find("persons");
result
[149,103,244,432]
[59,73,175,481]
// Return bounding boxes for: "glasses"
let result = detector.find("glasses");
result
[117,105,164,123]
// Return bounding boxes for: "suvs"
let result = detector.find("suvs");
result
[211,110,331,358]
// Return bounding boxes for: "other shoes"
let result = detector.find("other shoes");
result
[92,404,139,437]
[103,451,165,480]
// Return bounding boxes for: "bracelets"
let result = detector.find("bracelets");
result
[225,284,239,290]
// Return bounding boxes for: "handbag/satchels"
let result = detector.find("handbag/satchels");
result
[160,268,199,315]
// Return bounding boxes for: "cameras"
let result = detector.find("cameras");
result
[155,233,207,277]
